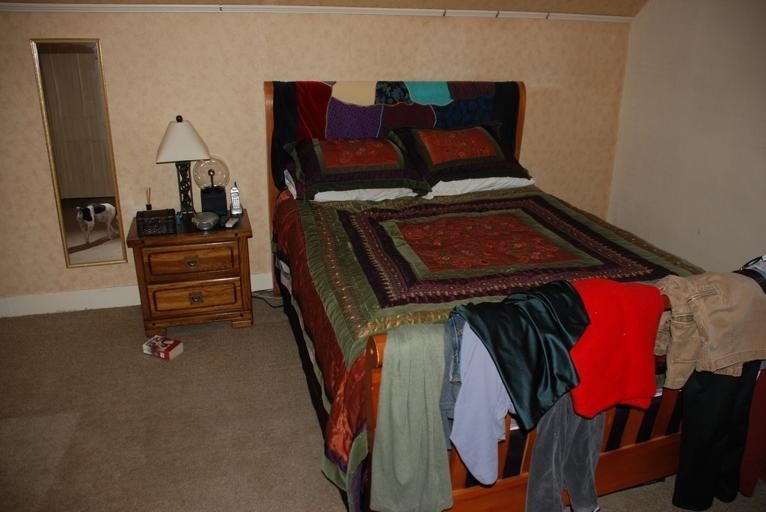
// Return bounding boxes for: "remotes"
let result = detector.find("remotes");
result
[224,216,239,230]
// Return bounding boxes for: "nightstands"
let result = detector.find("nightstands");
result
[126,208,253,338]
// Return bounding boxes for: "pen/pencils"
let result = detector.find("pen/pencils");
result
[145,188,152,203]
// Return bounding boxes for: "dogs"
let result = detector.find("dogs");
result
[76,202,120,245]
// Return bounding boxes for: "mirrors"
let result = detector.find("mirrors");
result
[30,37,128,269]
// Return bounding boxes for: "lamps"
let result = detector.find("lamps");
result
[155,116,210,210]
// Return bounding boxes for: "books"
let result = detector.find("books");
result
[143,335,184,361]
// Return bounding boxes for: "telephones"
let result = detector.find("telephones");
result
[230,182,243,216]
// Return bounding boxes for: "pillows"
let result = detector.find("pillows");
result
[280,136,434,203]
[390,124,535,197]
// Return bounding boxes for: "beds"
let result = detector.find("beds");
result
[263,80,766,512]
